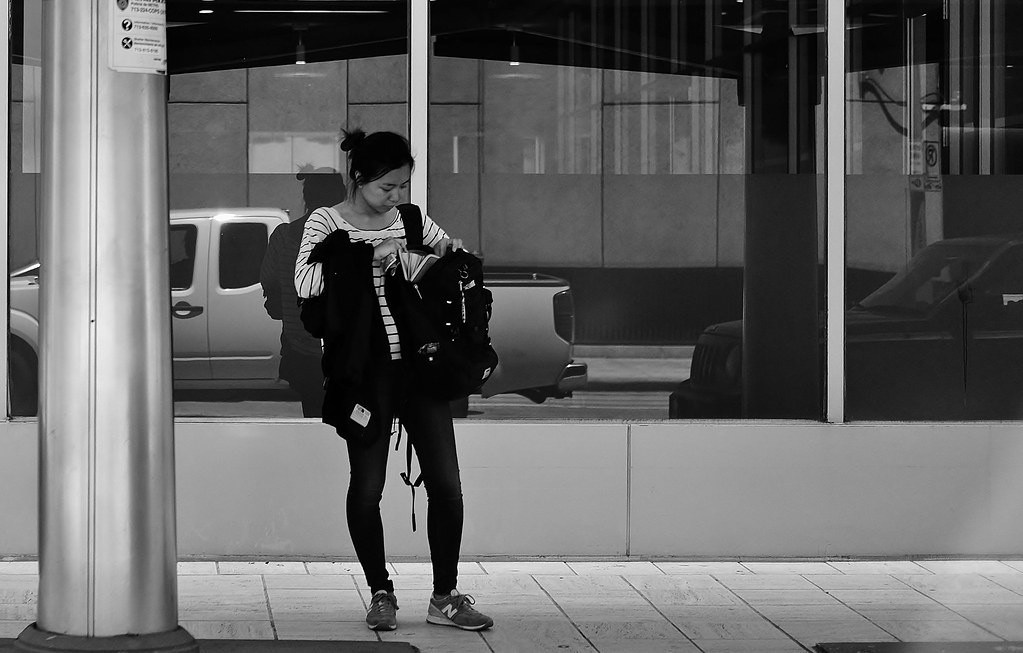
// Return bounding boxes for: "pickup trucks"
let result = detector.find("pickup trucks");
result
[9,206,590,419]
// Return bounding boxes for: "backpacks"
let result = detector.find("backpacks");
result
[384,203,499,404]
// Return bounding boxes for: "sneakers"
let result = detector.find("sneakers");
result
[426,588,493,630]
[365,590,399,630]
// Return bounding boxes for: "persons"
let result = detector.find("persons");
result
[293,127,494,631]
[259,163,349,418]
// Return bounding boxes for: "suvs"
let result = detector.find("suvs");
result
[668,234,1023,420]
